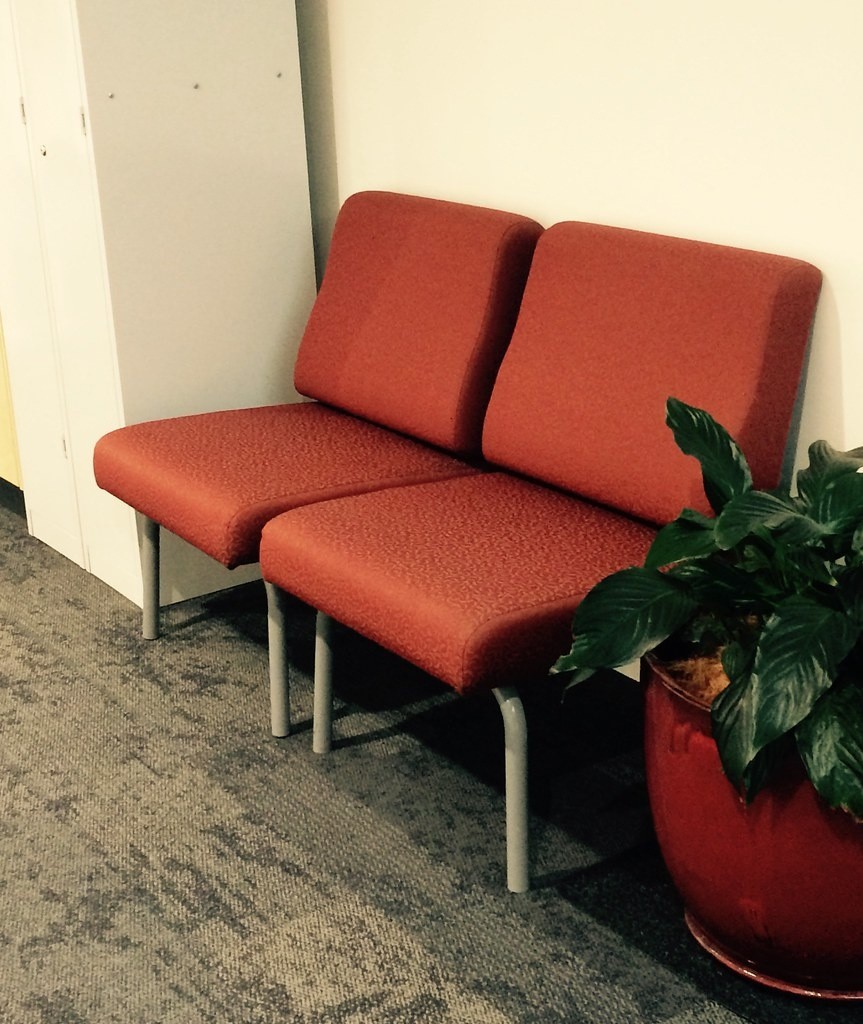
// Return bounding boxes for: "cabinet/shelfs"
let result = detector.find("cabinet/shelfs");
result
[4,1,319,610]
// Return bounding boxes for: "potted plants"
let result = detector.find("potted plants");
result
[547,392,863,1001]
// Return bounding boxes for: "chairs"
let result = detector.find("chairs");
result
[92,190,549,739]
[260,221,824,895]
